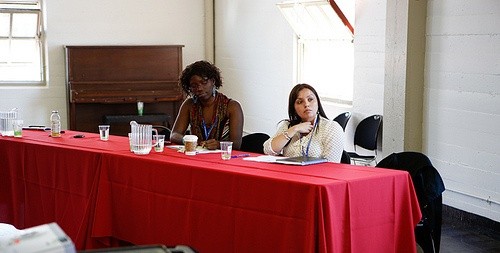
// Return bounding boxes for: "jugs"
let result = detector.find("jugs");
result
[0,112,16,136]
[132,124,158,155]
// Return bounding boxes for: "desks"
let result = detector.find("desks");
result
[0,128,422,253]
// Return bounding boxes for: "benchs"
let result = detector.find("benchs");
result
[102,112,170,132]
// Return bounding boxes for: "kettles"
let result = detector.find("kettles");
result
[13,120,23,137]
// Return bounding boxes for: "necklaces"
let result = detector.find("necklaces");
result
[202,118,218,141]
[299,114,318,157]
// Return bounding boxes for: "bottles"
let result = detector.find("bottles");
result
[50,109,60,137]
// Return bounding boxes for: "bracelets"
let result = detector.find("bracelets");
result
[282,130,291,140]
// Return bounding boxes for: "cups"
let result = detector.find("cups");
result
[129,133,135,151]
[220,142,233,160]
[153,135,165,152]
[99,126,110,140]
[183,135,198,155]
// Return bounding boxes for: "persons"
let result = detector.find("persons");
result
[263,84,344,164]
[169,60,244,152]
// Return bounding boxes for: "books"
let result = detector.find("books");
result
[276,156,328,166]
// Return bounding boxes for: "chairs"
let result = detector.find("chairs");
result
[151,126,171,141]
[332,112,446,253]
[239,132,270,155]
